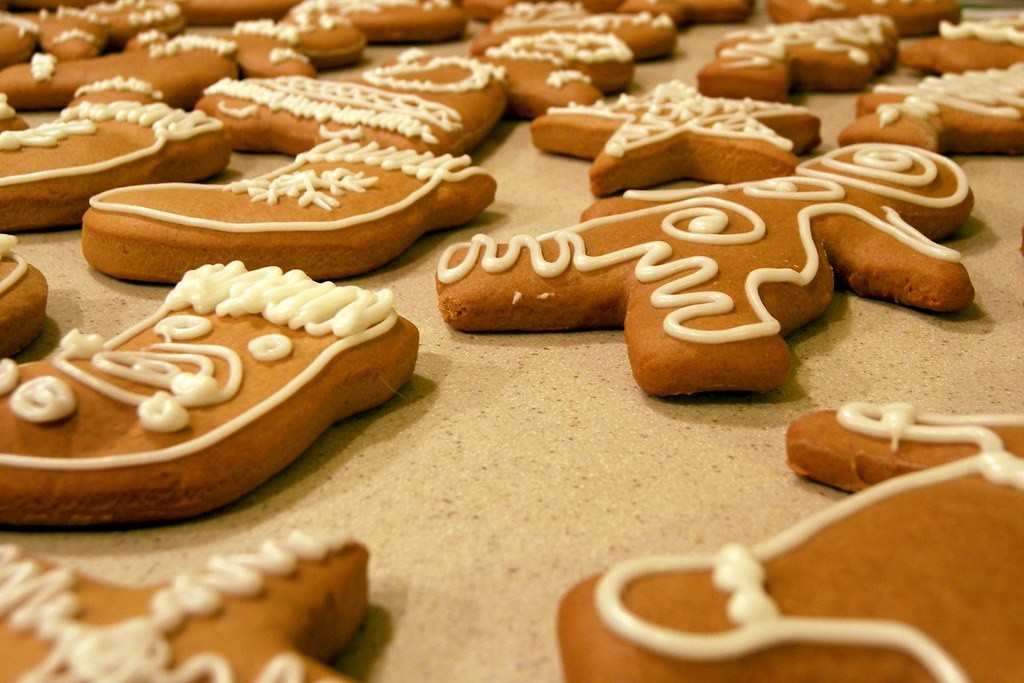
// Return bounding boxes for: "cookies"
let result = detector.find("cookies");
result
[0,0,1024,682]
